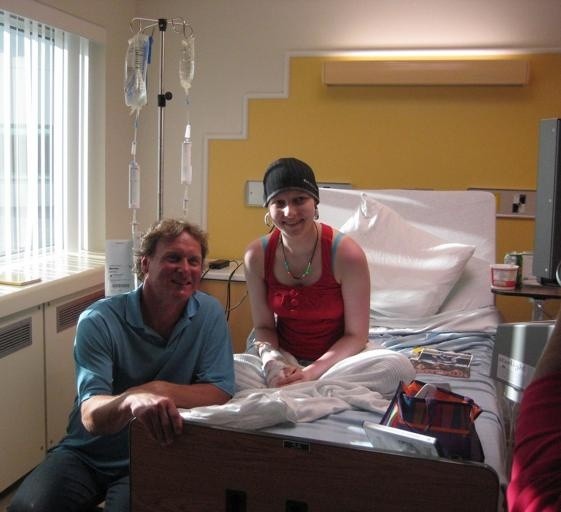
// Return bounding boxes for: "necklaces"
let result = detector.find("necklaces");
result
[280,220,320,281]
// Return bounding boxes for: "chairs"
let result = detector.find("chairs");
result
[489,322,558,477]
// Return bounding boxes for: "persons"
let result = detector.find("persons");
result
[223,155,419,396]
[5,215,238,512]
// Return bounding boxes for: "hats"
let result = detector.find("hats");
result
[262,156,322,207]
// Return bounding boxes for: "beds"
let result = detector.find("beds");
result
[128,188,508,512]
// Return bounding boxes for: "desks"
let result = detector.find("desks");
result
[489,281,561,321]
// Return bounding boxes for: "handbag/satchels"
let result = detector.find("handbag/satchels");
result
[377,379,488,463]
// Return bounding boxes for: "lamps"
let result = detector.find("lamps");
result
[322,57,530,86]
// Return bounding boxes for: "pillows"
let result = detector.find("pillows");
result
[336,194,475,320]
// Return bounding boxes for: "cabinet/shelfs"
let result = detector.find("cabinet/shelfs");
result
[0,282,110,494]
